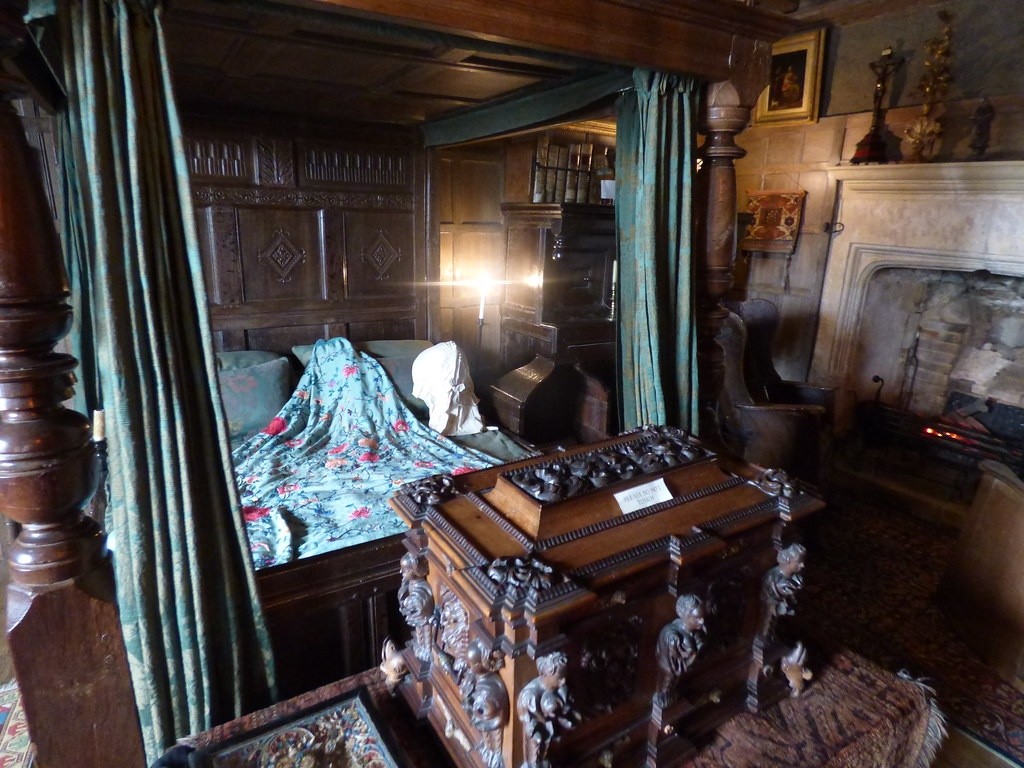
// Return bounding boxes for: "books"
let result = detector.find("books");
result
[528,133,615,205]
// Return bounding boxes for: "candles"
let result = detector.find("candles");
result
[478,292,486,320]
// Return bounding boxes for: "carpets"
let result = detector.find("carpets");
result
[685,498,1024,768]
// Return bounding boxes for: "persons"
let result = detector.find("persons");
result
[398,553,434,662]
[516,652,575,768]
[656,595,705,706]
[460,641,508,768]
[757,545,805,648]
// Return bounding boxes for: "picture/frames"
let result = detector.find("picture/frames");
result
[752,23,828,126]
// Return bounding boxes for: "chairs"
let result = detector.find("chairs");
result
[715,298,828,479]
[939,460,1024,686]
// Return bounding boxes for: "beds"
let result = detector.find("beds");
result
[18,0,802,768]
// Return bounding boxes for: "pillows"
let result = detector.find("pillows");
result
[215,337,436,436]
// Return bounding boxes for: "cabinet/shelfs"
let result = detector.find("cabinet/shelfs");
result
[499,199,617,383]
[380,422,823,768]
